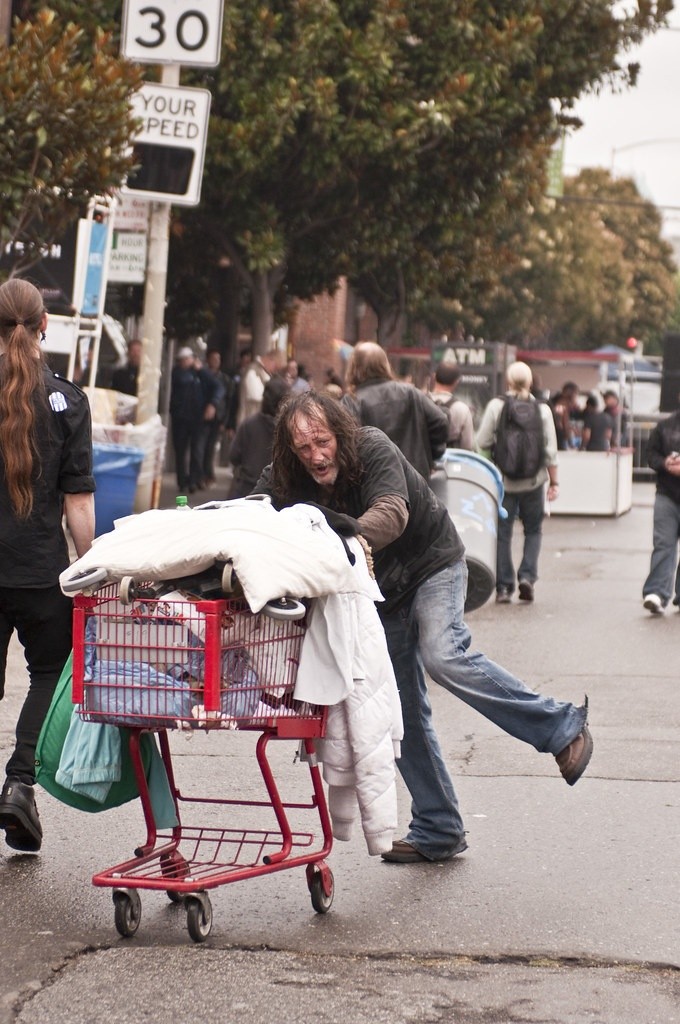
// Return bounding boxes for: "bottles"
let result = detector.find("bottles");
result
[174,495,192,511]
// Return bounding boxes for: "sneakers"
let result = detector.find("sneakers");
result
[0,775,43,852]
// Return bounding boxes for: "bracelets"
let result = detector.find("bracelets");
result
[550,481,558,485]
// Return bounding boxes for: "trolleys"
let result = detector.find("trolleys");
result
[73,578,335,941]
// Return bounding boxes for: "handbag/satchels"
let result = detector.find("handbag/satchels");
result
[34,644,150,814]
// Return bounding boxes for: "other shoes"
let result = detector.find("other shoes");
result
[643,593,665,614]
[555,727,593,786]
[381,828,470,862]
[518,579,533,601]
[497,586,509,602]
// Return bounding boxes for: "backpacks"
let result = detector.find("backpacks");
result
[491,393,544,481]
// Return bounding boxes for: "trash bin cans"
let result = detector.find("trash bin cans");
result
[91,442,145,539]
[437,448,508,613]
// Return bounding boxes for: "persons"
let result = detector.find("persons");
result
[112,341,141,397]
[252,388,596,862]
[172,349,309,499]
[327,342,445,478]
[477,362,559,601]
[641,410,680,615]
[429,360,474,450]
[0,278,95,852]
[548,391,628,450]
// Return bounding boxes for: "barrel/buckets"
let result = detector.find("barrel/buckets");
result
[92,440,144,537]
[430,446,509,614]
